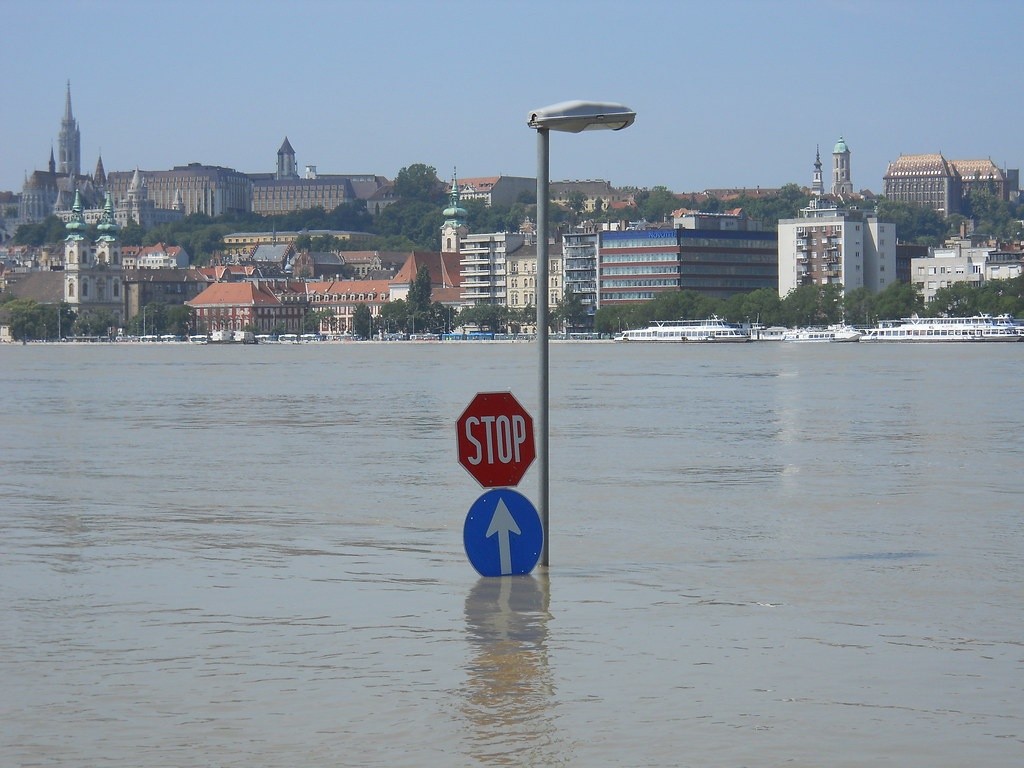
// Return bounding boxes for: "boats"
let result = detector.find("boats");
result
[752,326,787,340]
[612,318,751,343]
[856,316,1022,342]
[784,331,836,341]
[828,330,862,343]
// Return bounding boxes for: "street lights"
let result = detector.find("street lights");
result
[524,103,640,572]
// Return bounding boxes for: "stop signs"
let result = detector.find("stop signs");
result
[458,391,535,487]
